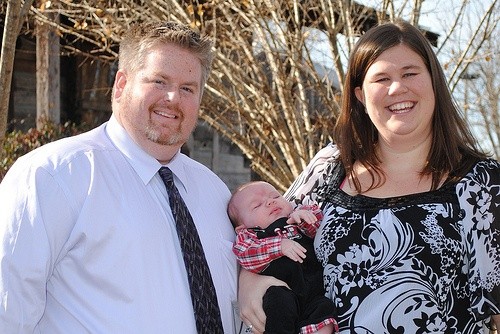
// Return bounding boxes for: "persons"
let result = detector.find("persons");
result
[226,181,338,334]
[238,19,500,334]
[0,21,250,334]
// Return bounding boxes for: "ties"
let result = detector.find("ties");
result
[157,166,224,333]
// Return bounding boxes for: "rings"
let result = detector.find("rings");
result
[248,325,254,329]
